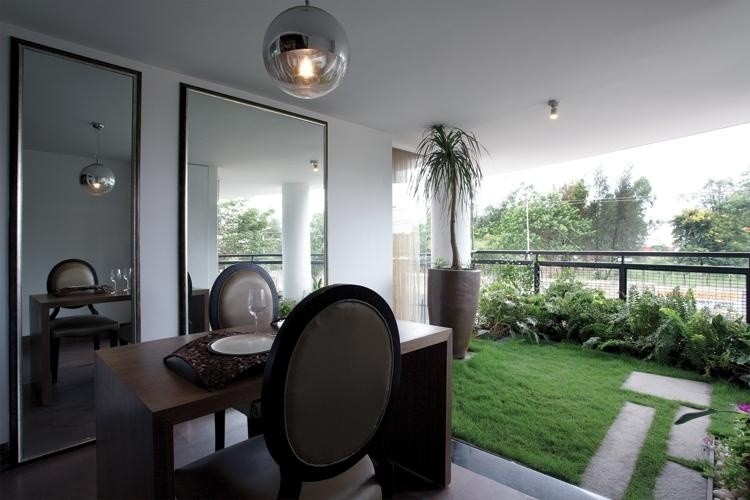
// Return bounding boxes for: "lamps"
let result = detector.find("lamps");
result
[548,98,561,119]
[263,0,350,101]
[79,121,116,195]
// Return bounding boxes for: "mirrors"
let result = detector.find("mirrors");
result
[180,81,328,337]
[8,34,142,469]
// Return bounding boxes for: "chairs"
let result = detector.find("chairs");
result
[46,257,119,384]
[210,262,280,452]
[173,284,401,500]
[118,321,134,345]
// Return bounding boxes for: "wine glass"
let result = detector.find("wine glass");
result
[122,266,134,292]
[110,268,122,294]
[248,289,268,339]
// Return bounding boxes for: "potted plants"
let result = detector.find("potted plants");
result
[409,123,492,361]
